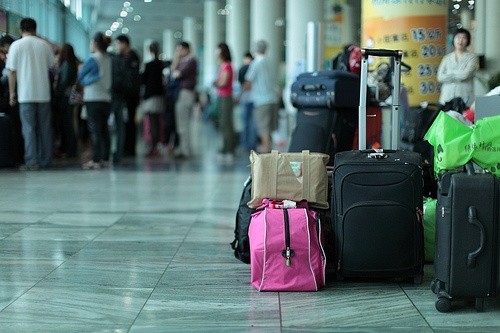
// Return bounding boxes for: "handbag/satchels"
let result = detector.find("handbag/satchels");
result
[425,109,500,177]
[231,176,253,265]
[69,81,84,106]
[247,201,328,293]
[249,148,332,211]
[335,42,477,150]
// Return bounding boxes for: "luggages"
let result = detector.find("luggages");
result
[288,107,358,167]
[332,47,425,286]
[430,159,500,313]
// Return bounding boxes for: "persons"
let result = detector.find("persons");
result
[435,28,479,107]
[0,17,282,172]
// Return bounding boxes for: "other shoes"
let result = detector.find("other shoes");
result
[99,160,113,168]
[82,160,101,170]
[19,164,38,172]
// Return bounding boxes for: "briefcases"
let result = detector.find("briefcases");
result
[289,70,361,108]
